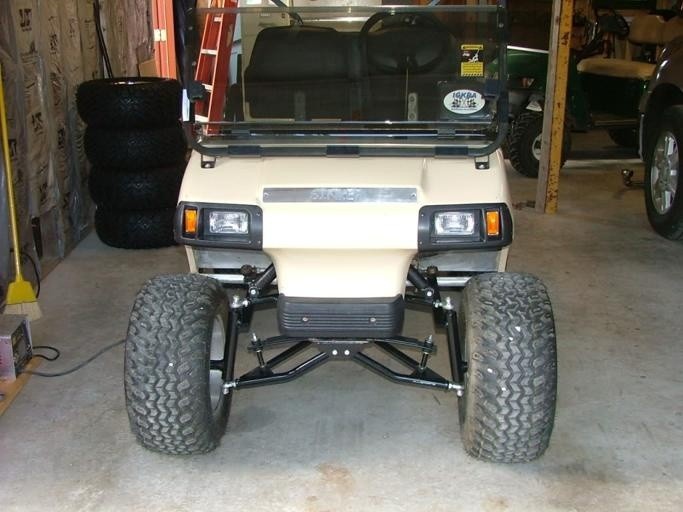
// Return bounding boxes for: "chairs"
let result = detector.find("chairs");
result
[225,24,461,121]
[574,9,681,161]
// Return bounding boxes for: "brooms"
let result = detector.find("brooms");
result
[0,65,44,323]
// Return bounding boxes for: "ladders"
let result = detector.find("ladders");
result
[194,0,238,134]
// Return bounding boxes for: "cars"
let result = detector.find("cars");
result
[637,36,683,244]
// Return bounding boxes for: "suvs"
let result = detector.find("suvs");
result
[123,0,558,465]
